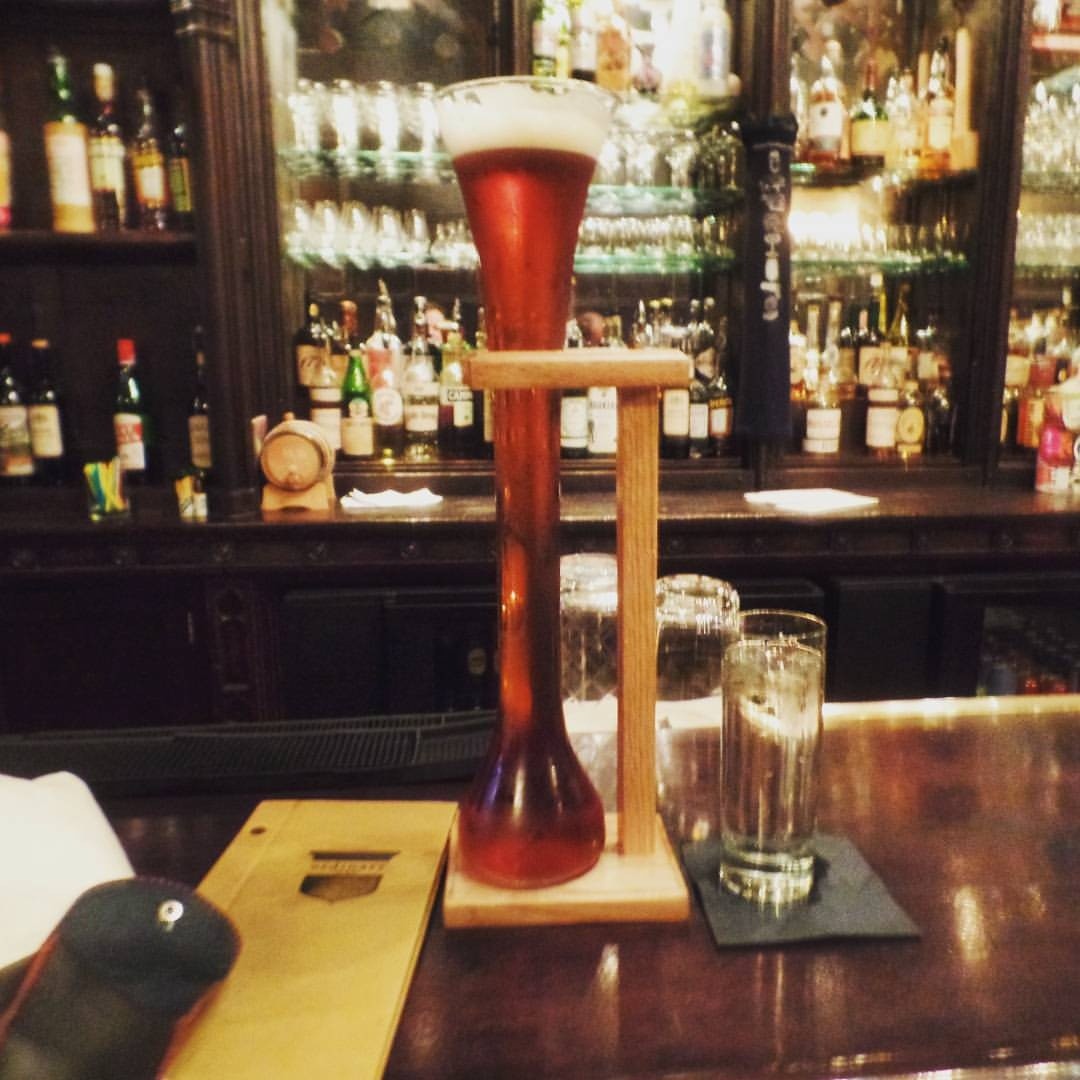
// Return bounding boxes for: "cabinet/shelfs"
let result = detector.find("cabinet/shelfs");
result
[1,0,1079,737]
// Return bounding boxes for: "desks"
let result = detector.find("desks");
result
[0,691,1080,1079]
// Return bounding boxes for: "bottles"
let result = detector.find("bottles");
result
[0,19,1080,523]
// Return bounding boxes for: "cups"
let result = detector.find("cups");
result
[655,574,744,843]
[721,608,827,908]
[553,551,622,815]
